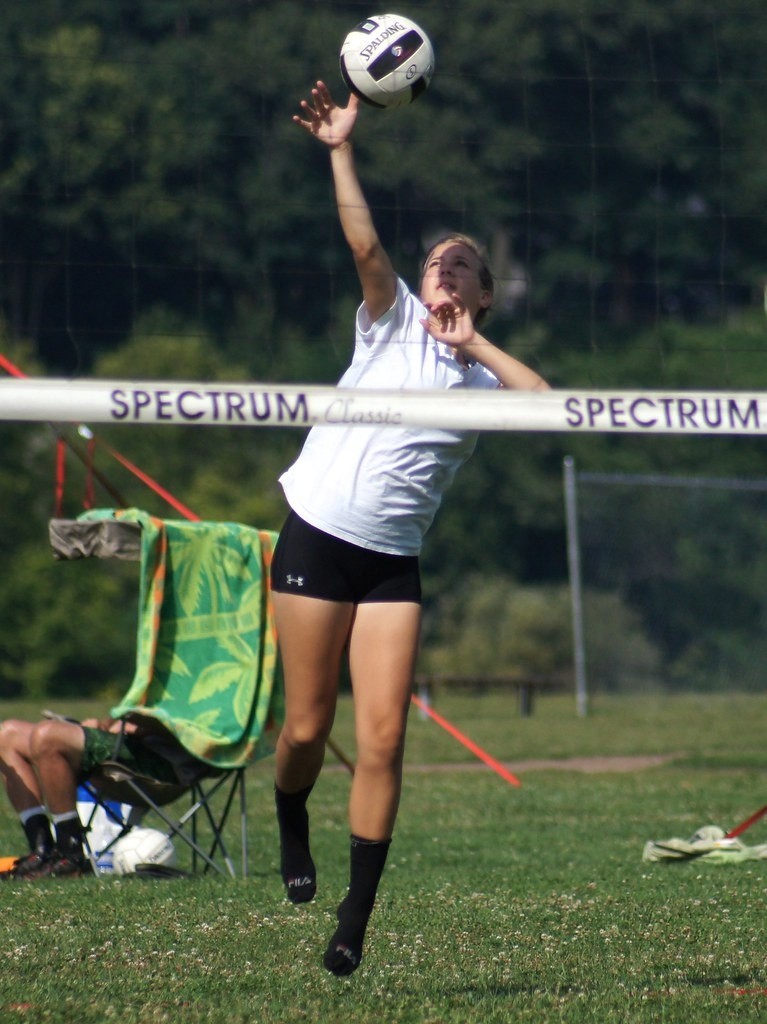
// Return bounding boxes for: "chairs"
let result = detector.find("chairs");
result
[48,510,280,880]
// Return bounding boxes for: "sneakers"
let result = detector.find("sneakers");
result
[23,850,95,882]
[0,851,42,879]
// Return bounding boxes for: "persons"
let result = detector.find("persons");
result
[0,713,219,881]
[272,76,556,980]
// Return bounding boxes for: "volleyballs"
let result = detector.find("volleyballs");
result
[114,828,176,876]
[339,12,436,109]
